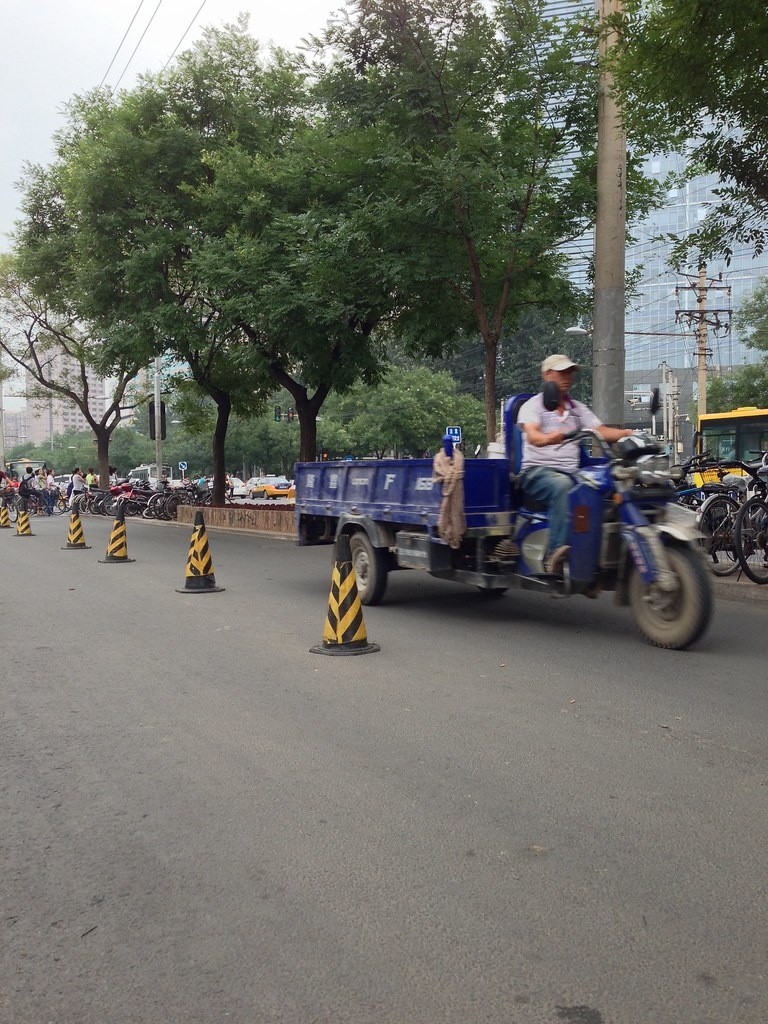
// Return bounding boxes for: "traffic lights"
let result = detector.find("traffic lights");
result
[322,448,328,461]
[274,406,282,422]
[287,407,295,422]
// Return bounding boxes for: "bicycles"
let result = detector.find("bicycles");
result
[0,479,234,522]
[664,448,768,584]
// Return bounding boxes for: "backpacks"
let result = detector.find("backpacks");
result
[18,475,34,497]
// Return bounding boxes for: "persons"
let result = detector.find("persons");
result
[516,355,634,574]
[722,444,735,461]
[109,466,117,481]
[199,475,209,504]
[225,474,235,500]
[67,468,98,513]
[183,476,190,486]
[0,466,19,512]
[20,467,56,517]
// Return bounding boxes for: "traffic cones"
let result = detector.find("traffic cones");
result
[0,497,13,529]
[61,501,94,550]
[170,513,227,594]
[97,502,135,565]
[306,533,383,655]
[10,496,37,536]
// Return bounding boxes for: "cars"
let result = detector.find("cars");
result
[249,473,292,500]
[245,477,262,492]
[225,477,249,500]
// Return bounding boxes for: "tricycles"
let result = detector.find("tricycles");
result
[290,390,716,654]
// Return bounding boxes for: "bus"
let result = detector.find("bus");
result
[128,463,174,488]
[687,405,768,492]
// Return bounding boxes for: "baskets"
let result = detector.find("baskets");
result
[2,490,14,499]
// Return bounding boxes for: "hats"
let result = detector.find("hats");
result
[541,354,579,372]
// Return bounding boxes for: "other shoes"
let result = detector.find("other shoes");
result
[230,497,235,500]
[547,544,571,573]
